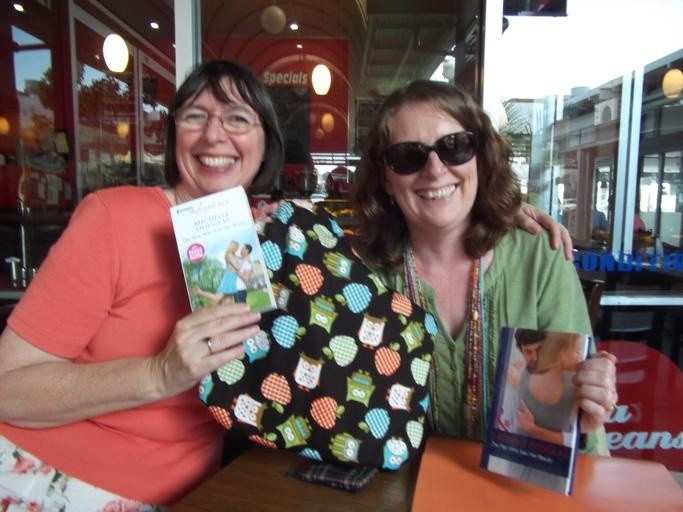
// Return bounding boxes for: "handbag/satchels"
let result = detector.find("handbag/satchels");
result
[194,196,437,475]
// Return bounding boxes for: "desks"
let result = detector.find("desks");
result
[168,434,683,511]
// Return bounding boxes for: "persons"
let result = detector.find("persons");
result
[0,59,572,512]
[509,328,572,448]
[634,207,645,233]
[590,208,606,244]
[348,78,617,458]
[192,241,240,306]
[504,332,583,437]
[229,243,252,303]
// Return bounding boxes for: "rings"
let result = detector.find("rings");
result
[606,404,613,412]
[204,338,213,354]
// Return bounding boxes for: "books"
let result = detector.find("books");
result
[409,436,682,512]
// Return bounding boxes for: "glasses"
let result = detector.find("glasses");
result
[169,105,266,133]
[379,131,478,175]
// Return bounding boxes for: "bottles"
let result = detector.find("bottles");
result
[29,265,39,280]
[6,260,22,289]
[19,266,30,289]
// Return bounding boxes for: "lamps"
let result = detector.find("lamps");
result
[260,0,287,34]
[661,64,683,102]
